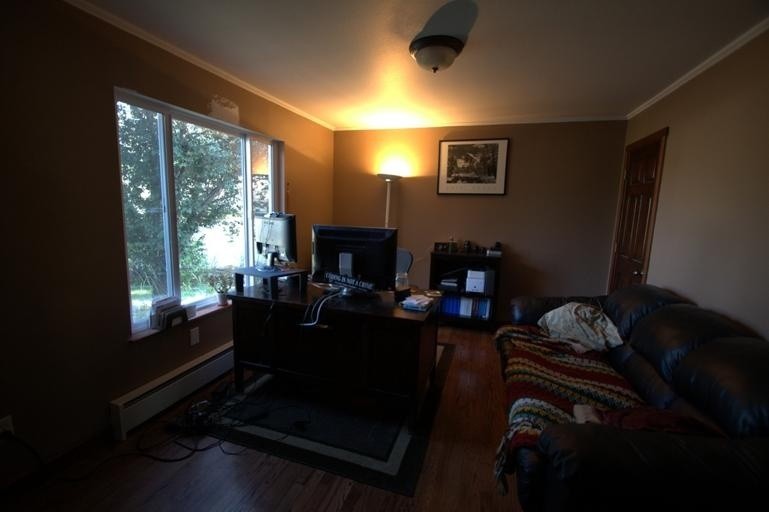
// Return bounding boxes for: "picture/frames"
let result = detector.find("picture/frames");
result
[434,242,449,254]
[438,138,510,195]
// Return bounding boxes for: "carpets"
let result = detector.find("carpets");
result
[186,342,457,497]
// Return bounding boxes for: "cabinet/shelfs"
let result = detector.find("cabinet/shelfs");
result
[429,251,503,334]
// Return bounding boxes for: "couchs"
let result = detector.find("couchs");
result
[495,283,769,512]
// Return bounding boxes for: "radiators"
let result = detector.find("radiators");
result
[108,340,235,442]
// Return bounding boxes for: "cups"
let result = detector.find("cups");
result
[463,239,486,253]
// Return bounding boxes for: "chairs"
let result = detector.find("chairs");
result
[395,247,413,286]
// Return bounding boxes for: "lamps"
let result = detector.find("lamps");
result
[411,36,465,73]
[377,158,409,229]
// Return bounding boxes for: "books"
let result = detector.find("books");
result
[149,296,196,329]
[440,292,487,319]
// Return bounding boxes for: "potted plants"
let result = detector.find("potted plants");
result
[207,271,234,306]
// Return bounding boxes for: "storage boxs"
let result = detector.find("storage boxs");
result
[465,269,502,298]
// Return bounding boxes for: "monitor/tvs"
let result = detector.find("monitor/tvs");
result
[254,210,298,274]
[311,223,399,302]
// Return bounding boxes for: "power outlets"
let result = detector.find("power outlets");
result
[0,413,16,437]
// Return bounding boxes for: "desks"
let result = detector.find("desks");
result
[226,277,445,412]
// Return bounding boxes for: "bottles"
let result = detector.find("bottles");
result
[395,271,410,303]
